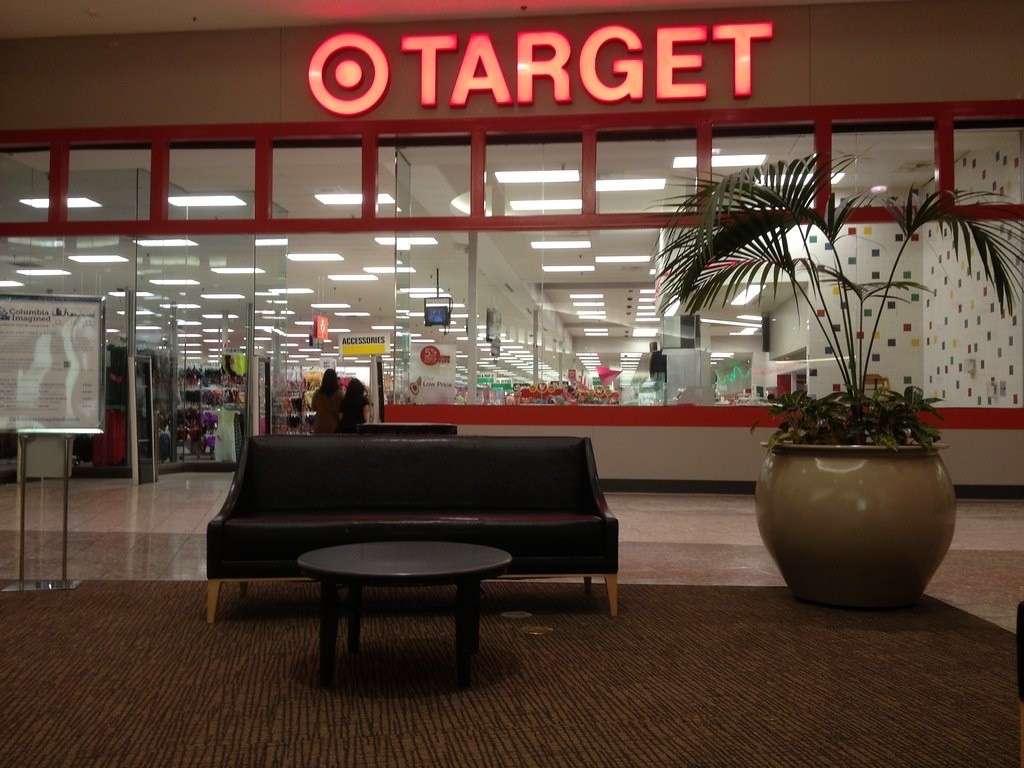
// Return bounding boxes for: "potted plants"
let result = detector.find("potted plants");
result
[645,145,1024,610]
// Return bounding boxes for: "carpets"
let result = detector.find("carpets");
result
[1,577,1024,768]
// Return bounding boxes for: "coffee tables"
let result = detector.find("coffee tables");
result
[296,541,514,691]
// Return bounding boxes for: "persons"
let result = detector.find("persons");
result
[311,369,344,436]
[336,378,370,434]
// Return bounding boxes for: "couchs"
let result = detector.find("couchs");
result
[207,434,620,624]
